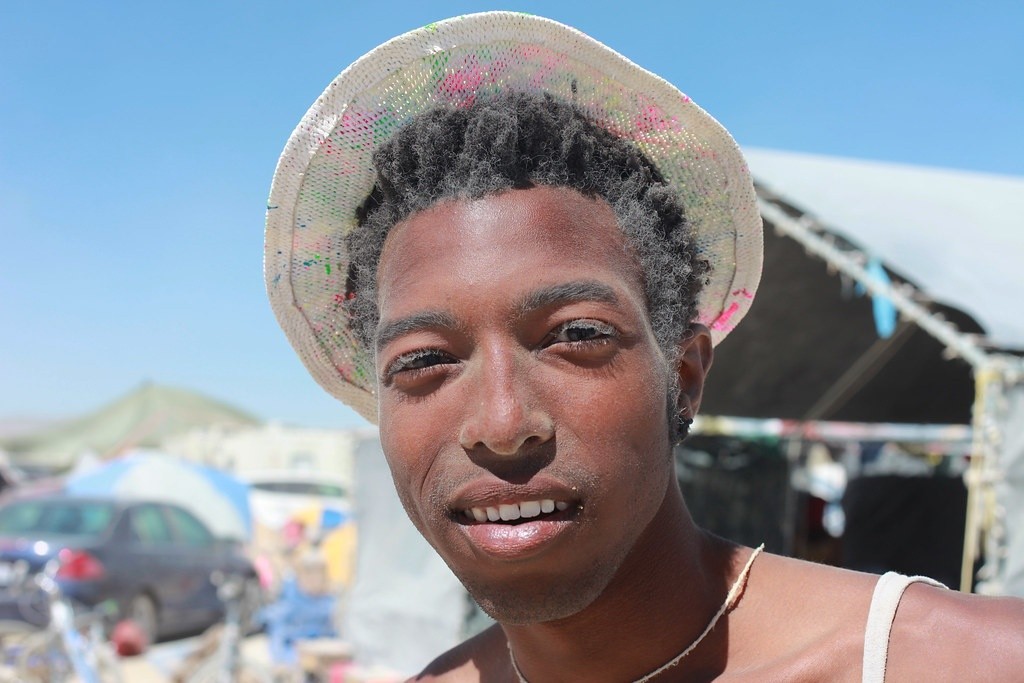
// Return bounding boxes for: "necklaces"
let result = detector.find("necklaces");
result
[502,539,771,683]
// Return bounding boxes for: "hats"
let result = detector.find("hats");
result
[264,10,765,426]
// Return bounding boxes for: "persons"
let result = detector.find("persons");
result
[211,518,334,683]
[263,12,1023,683]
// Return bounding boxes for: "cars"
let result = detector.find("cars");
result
[0,492,273,656]
